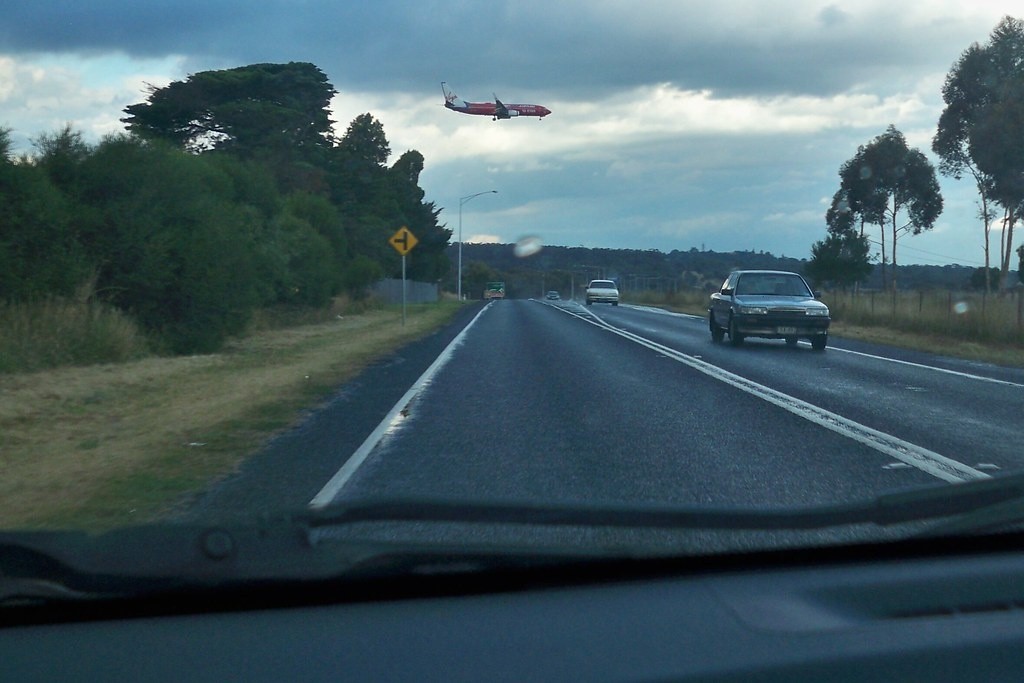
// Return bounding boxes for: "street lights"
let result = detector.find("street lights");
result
[459,190,498,300]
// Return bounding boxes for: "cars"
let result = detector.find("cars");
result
[709,269,831,350]
[546,291,560,299]
[584,279,620,306]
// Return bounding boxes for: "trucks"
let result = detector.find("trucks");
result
[483,281,504,299]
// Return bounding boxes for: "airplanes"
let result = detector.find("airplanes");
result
[439,82,551,121]
